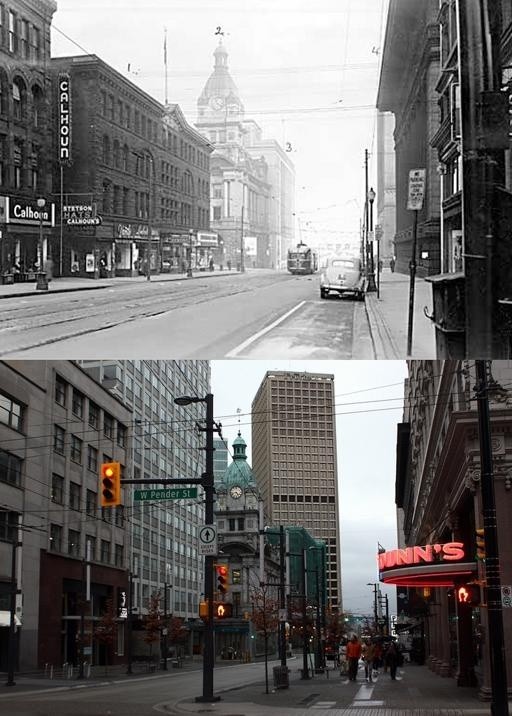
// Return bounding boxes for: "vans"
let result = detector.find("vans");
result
[321,643,338,661]
[339,639,348,655]
[359,636,371,651]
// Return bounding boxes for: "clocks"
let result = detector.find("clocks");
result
[230,486,242,499]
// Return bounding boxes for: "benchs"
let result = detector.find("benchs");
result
[298,668,334,678]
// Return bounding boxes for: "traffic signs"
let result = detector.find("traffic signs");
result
[134,487,200,501]
[196,523,219,555]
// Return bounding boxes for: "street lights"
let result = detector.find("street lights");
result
[127,570,140,675]
[33,193,48,290]
[7,539,25,688]
[367,186,377,292]
[186,228,193,276]
[173,394,222,700]
[77,560,92,679]
[258,524,328,683]
[366,583,389,636]
[162,582,173,672]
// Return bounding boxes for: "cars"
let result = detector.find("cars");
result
[217,647,240,660]
[319,254,369,302]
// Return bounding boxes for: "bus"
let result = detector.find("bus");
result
[286,243,318,274]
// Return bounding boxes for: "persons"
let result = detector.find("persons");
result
[390,257,395,273]
[345,634,407,683]
[378,258,384,272]
[208,257,241,272]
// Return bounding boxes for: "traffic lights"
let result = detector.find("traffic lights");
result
[98,462,122,508]
[217,564,228,594]
[212,602,233,621]
[199,601,209,623]
[454,584,479,608]
